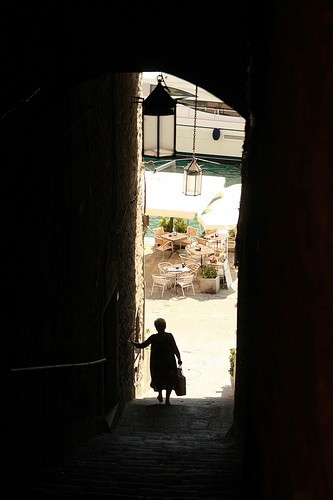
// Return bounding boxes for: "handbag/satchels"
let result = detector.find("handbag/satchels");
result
[174,363,186,396]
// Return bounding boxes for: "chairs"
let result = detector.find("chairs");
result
[151,226,236,299]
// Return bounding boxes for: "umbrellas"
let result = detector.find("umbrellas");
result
[199,183,242,236]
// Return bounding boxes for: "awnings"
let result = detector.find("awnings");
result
[142,171,226,219]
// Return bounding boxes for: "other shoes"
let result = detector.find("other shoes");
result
[165,400,171,405]
[157,396,163,402]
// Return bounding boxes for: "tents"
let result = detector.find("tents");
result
[175,104,245,157]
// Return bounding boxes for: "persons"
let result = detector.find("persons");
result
[128,318,182,406]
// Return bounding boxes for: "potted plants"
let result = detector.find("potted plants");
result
[198,265,220,293]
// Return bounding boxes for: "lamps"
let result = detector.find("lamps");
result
[142,71,177,160]
[182,85,202,197]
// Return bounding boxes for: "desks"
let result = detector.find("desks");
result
[188,247,215,266]
[161,232,187,259]
[204,234,224,248]
[167,267,190,295]
